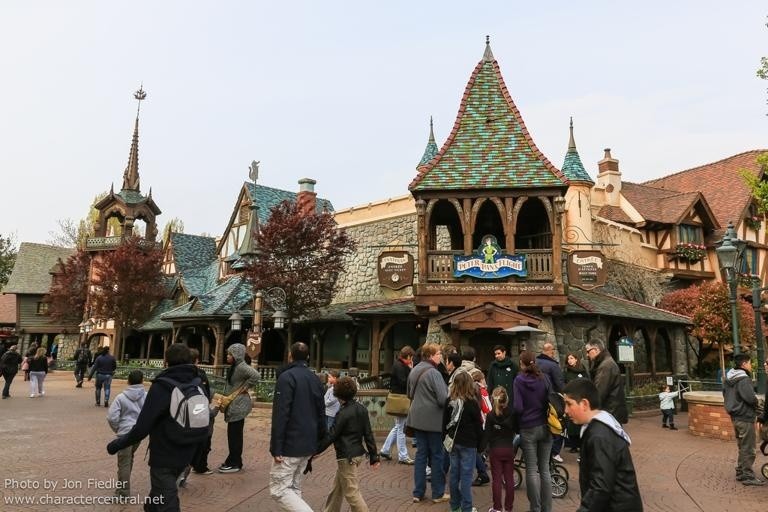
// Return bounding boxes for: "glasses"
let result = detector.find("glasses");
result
[586,346,597,354]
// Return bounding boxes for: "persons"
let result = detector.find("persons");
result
[658,385,679,430]
[379,338,645,512]
[269,342,328,512]
[311,376,381,512]
[0,337,58,399]
[323,369,342,432]
[68,342,259,512]
[480,238,497,276]
[723,352,768,485]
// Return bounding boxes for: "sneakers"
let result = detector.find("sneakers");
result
[487,507,502,512]
[97,399,109,408]
[190,466,214,475]
[471,506,478,512]
[551,454,563,462]
[377,450,392,460]
[412,495,427,503]
[30,390,46,398]
[430,493,451,503]
[396,457,415,465]
[75,380,83,388]
[217,459,242,473]
[734,469,766,487]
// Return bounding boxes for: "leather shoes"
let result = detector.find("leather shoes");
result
[469,474,490,487]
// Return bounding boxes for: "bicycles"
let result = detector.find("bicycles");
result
[761,463,768,479]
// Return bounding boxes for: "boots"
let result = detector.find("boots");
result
[662,423,669,428]
[669,423,679,430]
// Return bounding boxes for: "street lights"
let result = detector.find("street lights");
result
[715,220,748,355]
[229,286,289,407]
[78,317,94,340]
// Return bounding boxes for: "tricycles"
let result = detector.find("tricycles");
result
[511,444,569,498]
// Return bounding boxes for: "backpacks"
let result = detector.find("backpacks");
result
[151,368,212,432]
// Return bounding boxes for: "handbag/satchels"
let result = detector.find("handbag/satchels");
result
[538,369,565,436]
[402,414,415,438]
[442,433,456,454]
[210,391,230,413]
